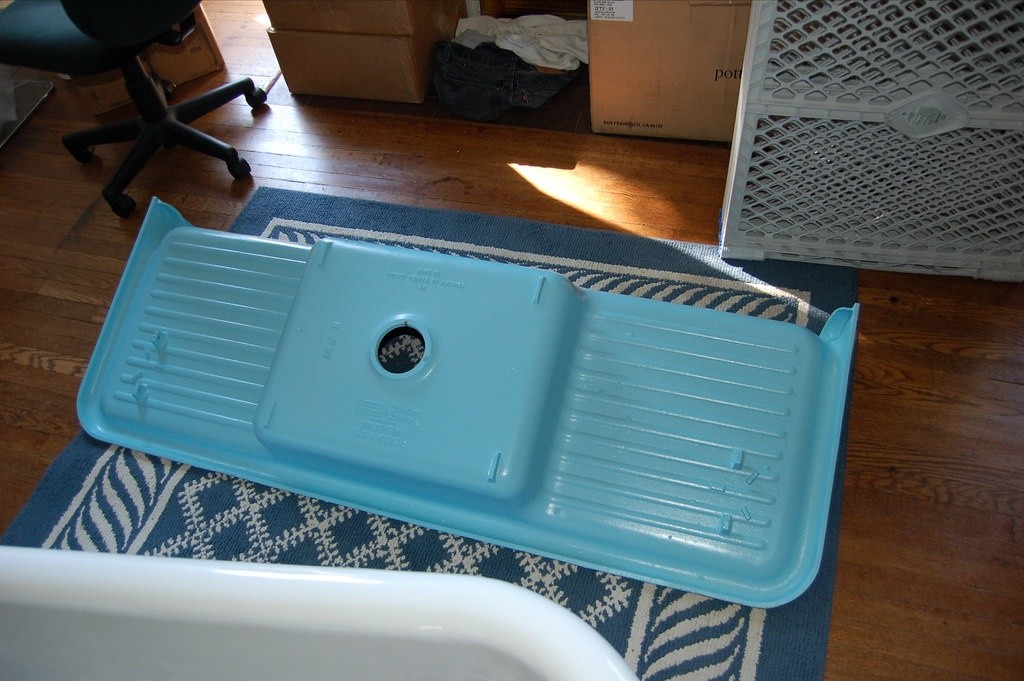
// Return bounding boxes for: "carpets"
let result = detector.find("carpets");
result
[0,183,858,681]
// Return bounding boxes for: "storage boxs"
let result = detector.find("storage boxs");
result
[262,0,829,144]
[69,4,226,117]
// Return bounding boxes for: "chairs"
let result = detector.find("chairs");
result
[0,0,269,218]
